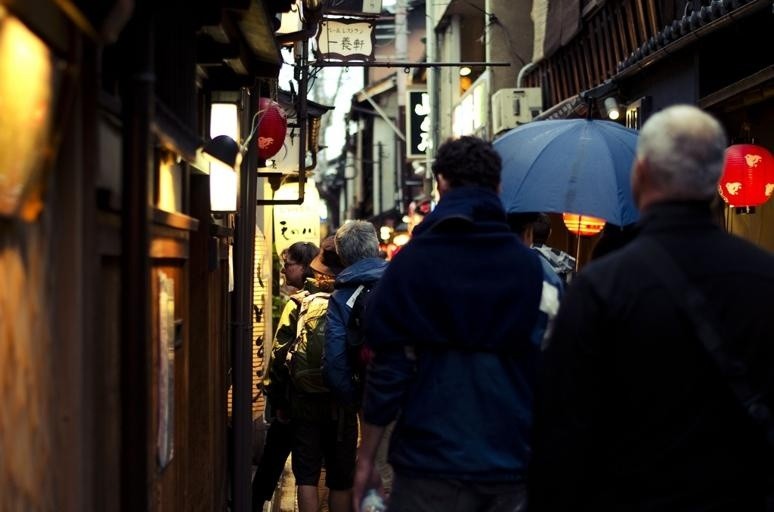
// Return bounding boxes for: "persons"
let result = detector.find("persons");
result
[351,137,544,510]
[250,209,575,511]
[539,103,772,511]
[502,211,562,349]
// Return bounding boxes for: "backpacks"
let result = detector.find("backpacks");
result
[286,295,331,400]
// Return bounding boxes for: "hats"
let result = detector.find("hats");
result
[309,236,341,278]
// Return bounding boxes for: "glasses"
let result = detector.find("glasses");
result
[284,262,297,269]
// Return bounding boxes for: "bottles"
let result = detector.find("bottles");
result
[361,488,386,512]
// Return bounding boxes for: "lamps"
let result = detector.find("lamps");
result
[602,97,632,121]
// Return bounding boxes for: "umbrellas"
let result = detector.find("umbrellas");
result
[489,97,644,228]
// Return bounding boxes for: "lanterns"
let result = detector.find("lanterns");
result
[562,212,607,237]
[716,144,773,213]
[258,97,287,159]
